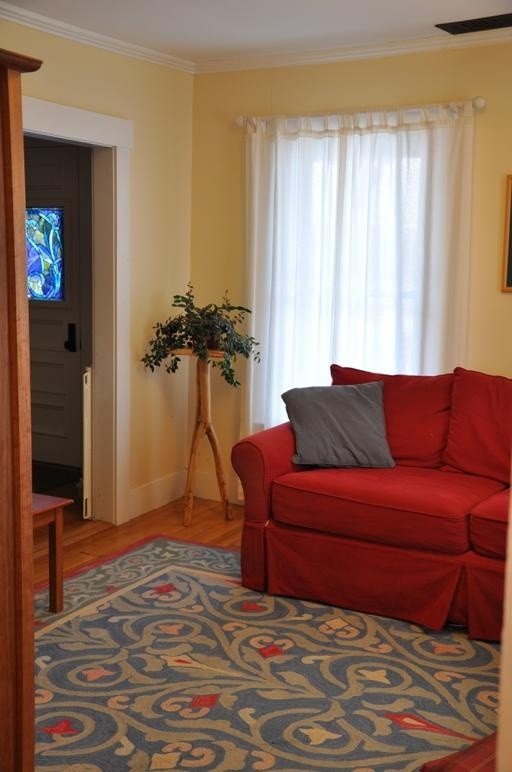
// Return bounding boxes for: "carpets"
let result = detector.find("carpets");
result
[29,535,497,772]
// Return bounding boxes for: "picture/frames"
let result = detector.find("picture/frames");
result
[497,174,512,294]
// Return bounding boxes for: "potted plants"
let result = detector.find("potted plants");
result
[139,280,262,389]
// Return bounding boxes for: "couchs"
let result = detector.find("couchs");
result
[229,363,512,642]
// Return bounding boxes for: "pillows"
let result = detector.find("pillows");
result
[277,380,397,470]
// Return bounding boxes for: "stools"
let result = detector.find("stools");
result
[32,493,73,611]
